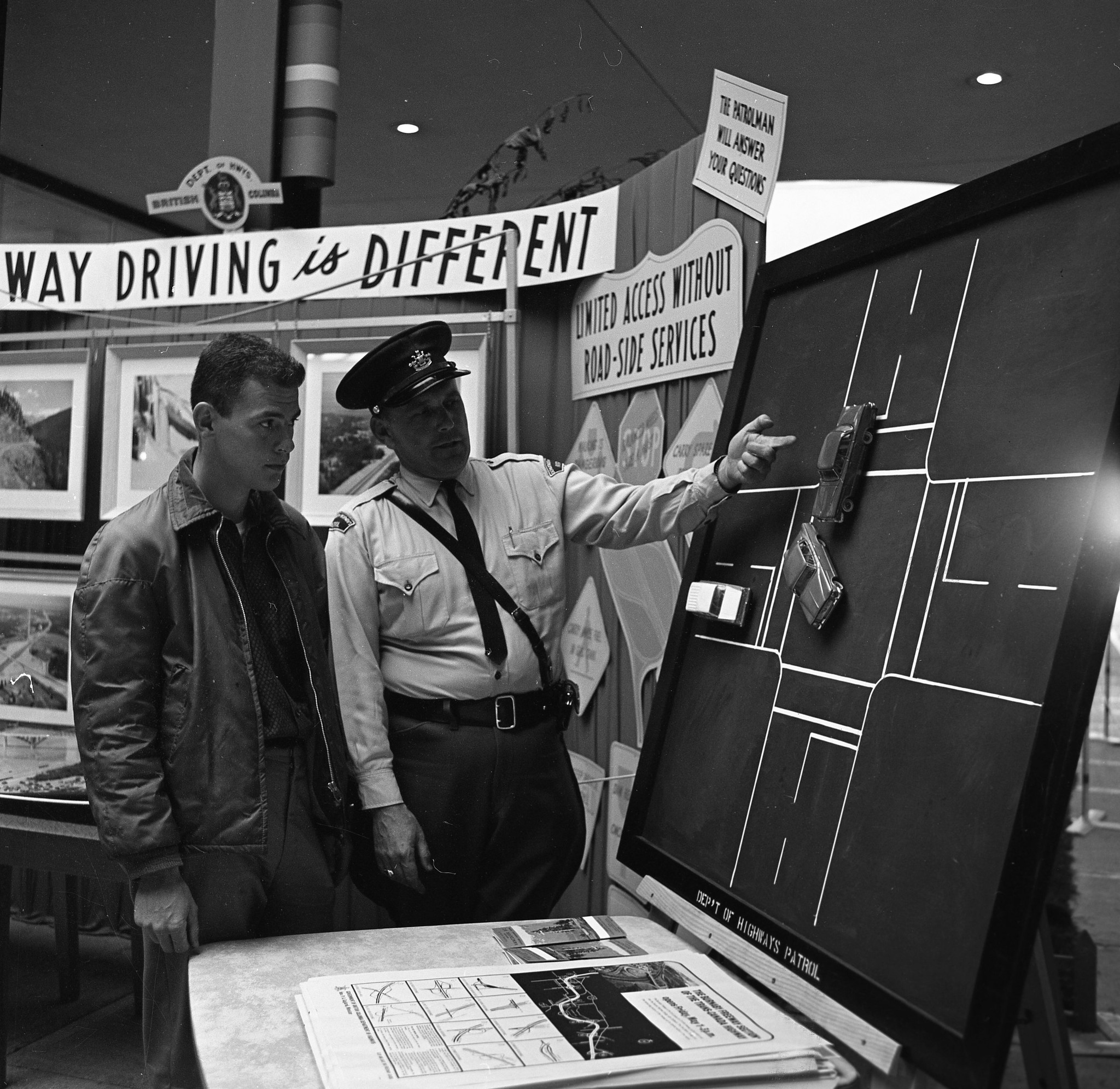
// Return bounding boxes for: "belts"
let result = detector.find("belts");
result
[383,689,563,731]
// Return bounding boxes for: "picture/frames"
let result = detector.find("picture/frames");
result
[99,340,274,520]
[283,332,489,528]
[0,348,90,522]
[0,568,81,727]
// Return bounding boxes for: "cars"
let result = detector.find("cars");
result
[685,578,754,630]
[809,400,880,523]
[783,522,845,632]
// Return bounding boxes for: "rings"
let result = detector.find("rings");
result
[385,869,393,877]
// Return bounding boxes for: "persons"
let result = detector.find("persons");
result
[324,322,797,926]
[70,332,351,1089]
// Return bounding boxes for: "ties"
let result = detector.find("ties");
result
[441,478,508,665]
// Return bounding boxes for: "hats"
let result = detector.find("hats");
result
[336,321,470,416]
